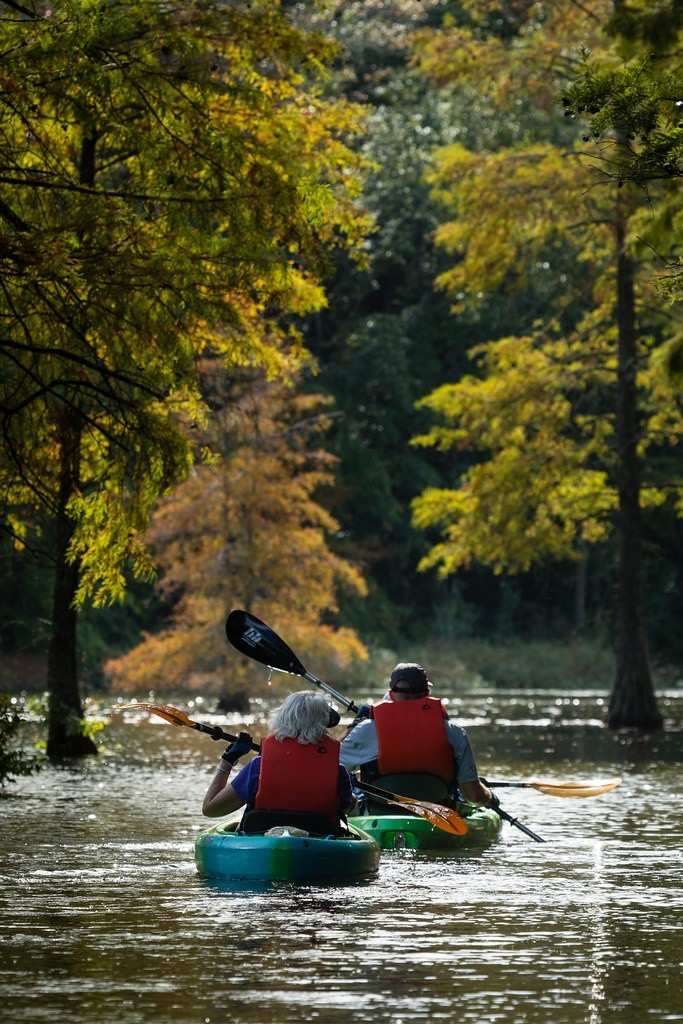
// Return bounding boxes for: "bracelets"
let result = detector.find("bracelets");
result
[217,768,230,775]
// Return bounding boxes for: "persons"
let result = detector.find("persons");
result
[202,690,353,839]
[339,661,500,820]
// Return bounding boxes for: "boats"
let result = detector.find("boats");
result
[347,799,504,852]
[194,813,382,884]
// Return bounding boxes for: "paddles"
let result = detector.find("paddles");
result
[112,699,471,840]
[223,604,546,845]
[476,774,628,800]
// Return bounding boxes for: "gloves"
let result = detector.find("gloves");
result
[222,732,253,766]
[486,792,500,810]
[349,772,357,789]
[347,705,370,729]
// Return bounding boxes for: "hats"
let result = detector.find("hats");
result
[390,663,433,694]
